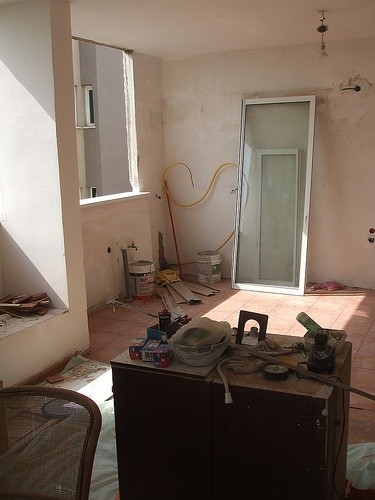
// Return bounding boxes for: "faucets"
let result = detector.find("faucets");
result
[127,241,138,252]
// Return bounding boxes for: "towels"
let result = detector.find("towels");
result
[156,269,181,287]
[308,280,344,292]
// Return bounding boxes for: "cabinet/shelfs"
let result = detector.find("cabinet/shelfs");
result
[110,330,352,500]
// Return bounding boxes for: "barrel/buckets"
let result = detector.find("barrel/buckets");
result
[195,250,222,283]
[128,260,155,299]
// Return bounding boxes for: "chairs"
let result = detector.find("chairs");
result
[0,386,102,500]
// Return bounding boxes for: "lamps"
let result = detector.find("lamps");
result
[316,10,330,58]
